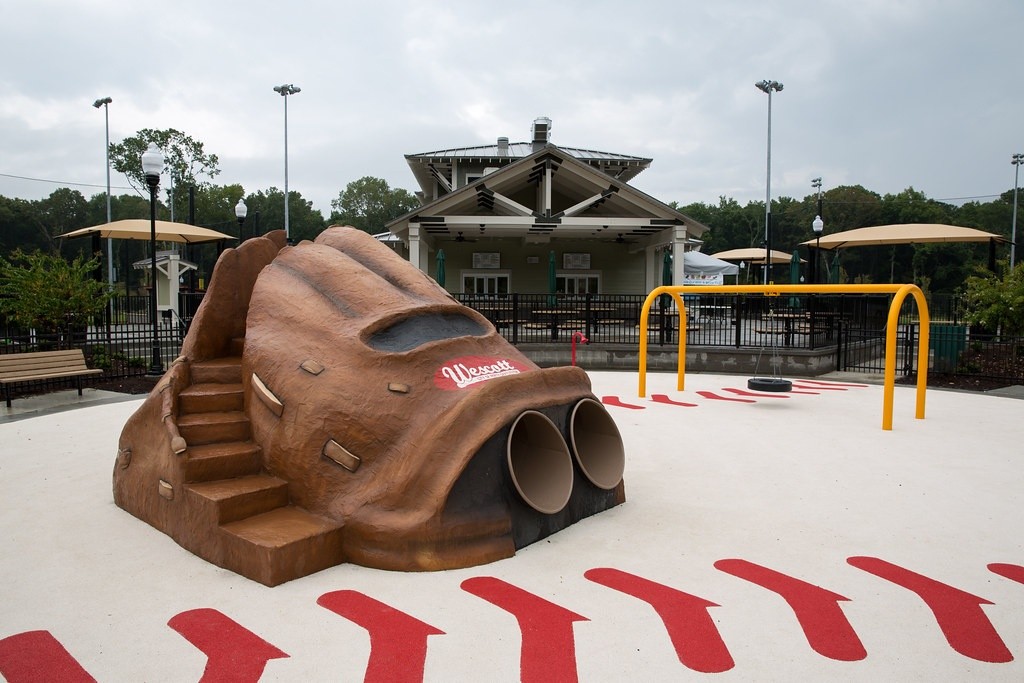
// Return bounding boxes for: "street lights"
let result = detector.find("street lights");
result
[92,96,115,324]
[235,199,248,244]
[1006,153,1024,296]
[739,260,745,284]
[810,176,823,219]
[811,215,824,284]
[140,141,165,375]
[273,84,301,247]
[754,79,784,314]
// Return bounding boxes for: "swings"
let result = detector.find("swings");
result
[747,296,793,393]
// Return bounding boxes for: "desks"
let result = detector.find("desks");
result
[806,312,840,340]
[762,314,810,345]
[532,311,579,340]
[477,308,513,334]
[648,312,688,342]
[575,308,617,333]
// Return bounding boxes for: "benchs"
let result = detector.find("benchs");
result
[635,325,660,332]
[674,326,702,332]
[0,349,103,407]
[497,320,586,330]
[597,320,624,325]
[753,325,838,335]
